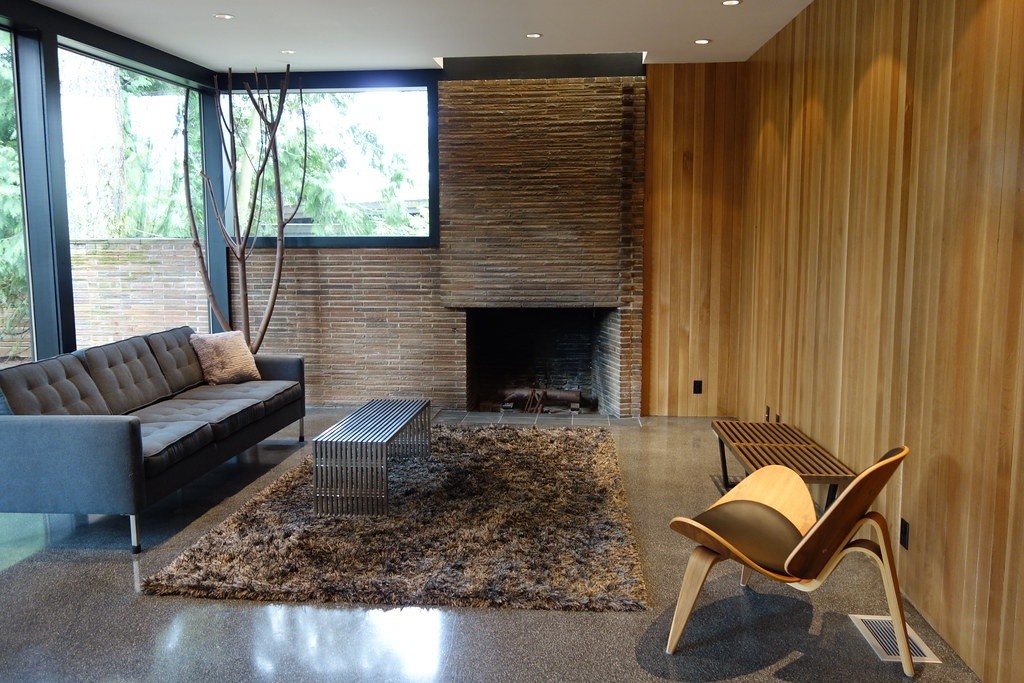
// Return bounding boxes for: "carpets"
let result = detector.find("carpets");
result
[142,424,651,613]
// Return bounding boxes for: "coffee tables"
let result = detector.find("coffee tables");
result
[311,399,433,516]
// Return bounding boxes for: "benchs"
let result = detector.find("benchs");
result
[0,325,307,554]
[707,420,858,507]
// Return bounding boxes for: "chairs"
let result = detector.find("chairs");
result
[665,445,915,678]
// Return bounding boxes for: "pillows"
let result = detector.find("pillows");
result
[190,331,261,386]
